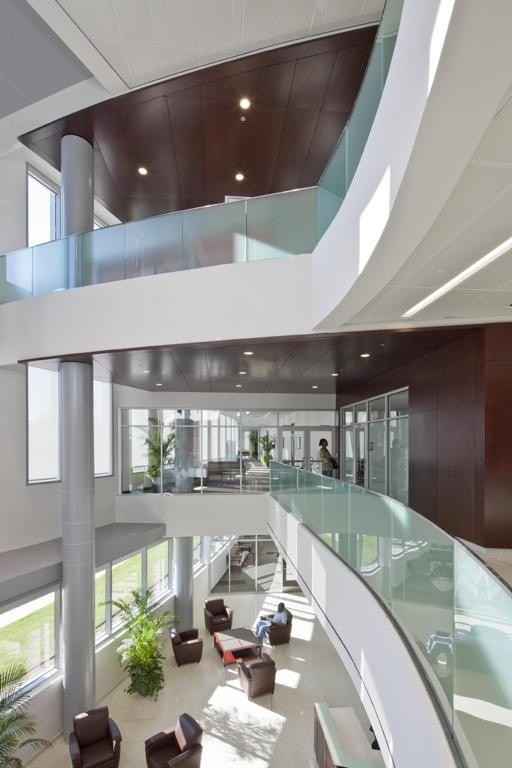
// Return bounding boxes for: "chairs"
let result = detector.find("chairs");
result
[260,608,293,645]
[203,598,233,634]
[237,653,276,702]
[170,628,203,667]
[145,713,203,768]
[68,706,123,768]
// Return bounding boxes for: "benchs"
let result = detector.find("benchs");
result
[207,461,245,482]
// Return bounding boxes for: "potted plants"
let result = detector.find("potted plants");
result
[99,584,181,701]
[138,416,176,493]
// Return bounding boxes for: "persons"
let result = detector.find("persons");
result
[390,439,402,498]
[254,602,287,639]
[319,438,337,477]
